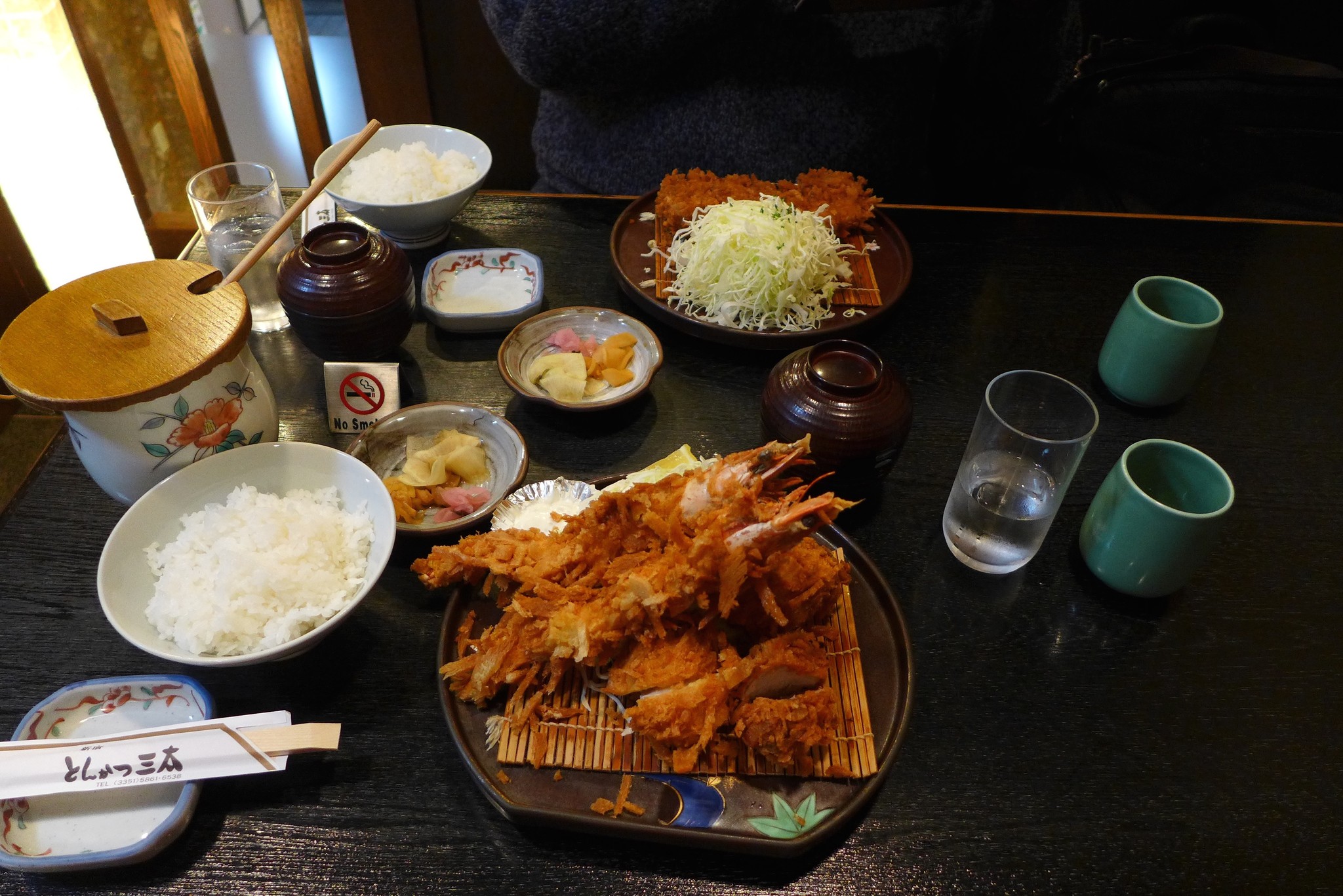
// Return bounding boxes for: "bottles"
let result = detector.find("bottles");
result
[759,339,910,510]
[276,221,414,362]
[62,343,279,505]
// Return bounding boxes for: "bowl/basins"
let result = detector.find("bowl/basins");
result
[97,442,397,668]
[343,401,528,533]
[497,306,663,410]
[313,124,492,249]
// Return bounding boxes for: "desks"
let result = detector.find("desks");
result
[0,185,1343,896]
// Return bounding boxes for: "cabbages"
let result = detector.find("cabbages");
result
[638,193,877,332]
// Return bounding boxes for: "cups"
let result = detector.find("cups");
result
[942,370,1100,572]
[186,162,297,332]
[1098,274,1224,411]
[1078,437,1236,598]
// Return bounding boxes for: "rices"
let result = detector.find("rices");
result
[340,141,482,203]
[142,484,376,657]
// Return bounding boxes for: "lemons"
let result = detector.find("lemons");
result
[605,443,699,494]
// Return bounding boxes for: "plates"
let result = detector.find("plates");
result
[438,475,914,850]
[609,187,912,336]
[0,673,212,873]
[420,248,545,332]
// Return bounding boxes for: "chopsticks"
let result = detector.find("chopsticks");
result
[238,722,341,757]
